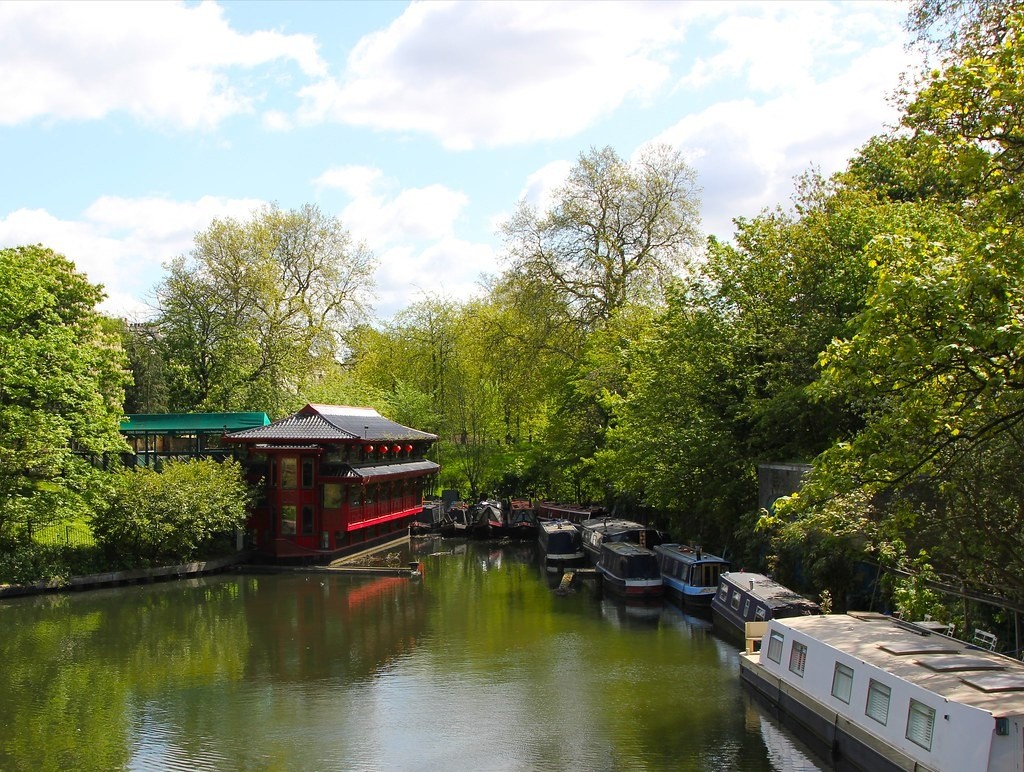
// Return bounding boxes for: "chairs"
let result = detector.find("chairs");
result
[924,614,933,621]
[947,623,956,637]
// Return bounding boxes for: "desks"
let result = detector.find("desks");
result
[913,621,949,634]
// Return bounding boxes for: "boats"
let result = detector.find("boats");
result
[738,609,1024,772]
[409,487,608,537]
[710,570,821,642]
[538,519,587,566]
[579,516,662,570]
[596,542,665,594]
[652,542,731,610]
